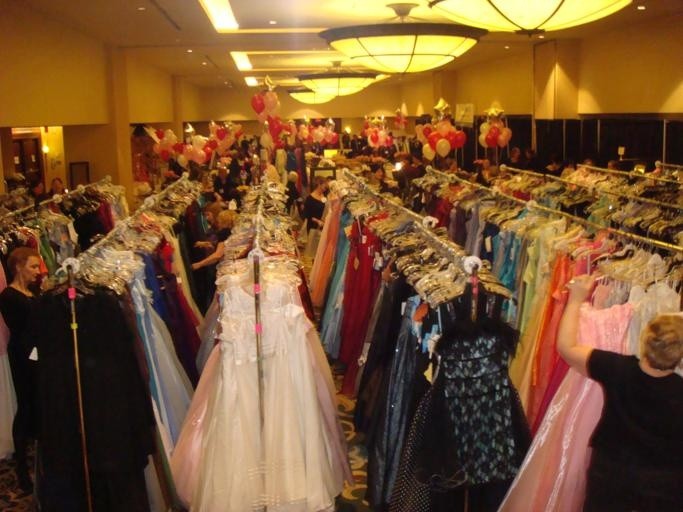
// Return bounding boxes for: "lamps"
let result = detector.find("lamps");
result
[427,0,633,38]
[319,3,489,75]
[298,61,377,97]
[287,88,337,106]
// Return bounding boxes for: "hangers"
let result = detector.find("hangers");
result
[511,164,683,284]
[56,178,204,295]
[215,178,303,290]
[0,177,123,254]
[330,170,511,305]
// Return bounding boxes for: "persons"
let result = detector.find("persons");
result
[504,147,523,170]
[302,176,330,264]
[47,179,67,200]
[193,203,224,254]
[249,147,261,181]
[192,211,237,296]
[406,152,426,182]
[201,183,223,203]
[0,247,61,491]
[241,135,250,150]
[285,170,301,214]
[443,158,457,176]
[322,175,335,197]
[214,167,237,201]
[555,273,683,511]
[367,164,390,196]
[27,181,49,204]
[523,150,542,173]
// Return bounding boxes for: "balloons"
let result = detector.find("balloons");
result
[474,119,511,149]
[148,117,245,170]
[394,117,408,129]
[250,89,343,149]
[410,120,466,161]
[360,122,394,148]
